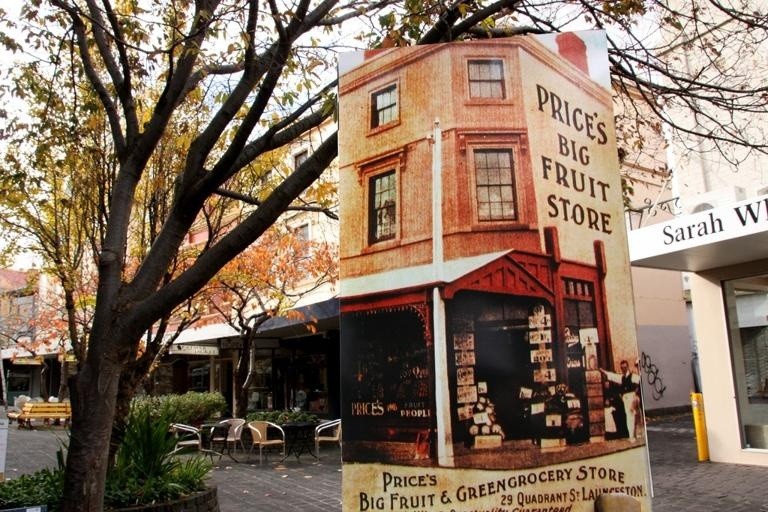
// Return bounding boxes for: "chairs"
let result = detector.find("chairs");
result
[167,417,341,468]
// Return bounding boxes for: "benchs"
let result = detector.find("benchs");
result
[7,401,70,433]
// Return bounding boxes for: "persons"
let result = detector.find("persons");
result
[599,360,643,443]
